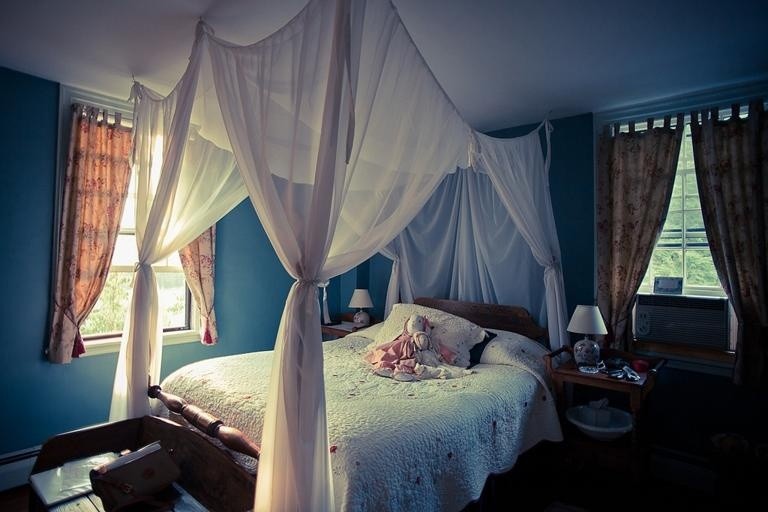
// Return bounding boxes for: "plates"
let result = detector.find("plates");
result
[577,365,599,374]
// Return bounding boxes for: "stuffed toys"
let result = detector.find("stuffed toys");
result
[363,314,458,381]
[411,331,474,380]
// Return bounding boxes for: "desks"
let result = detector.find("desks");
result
[536,341,675,445]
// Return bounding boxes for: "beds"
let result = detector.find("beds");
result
[140,292,562,511]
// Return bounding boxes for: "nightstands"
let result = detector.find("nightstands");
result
[316,313,365,341]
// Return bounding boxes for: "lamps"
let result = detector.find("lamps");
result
[348,285,375,327]
[566,303,606,368]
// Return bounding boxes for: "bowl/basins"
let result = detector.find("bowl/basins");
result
[564,405,633,442]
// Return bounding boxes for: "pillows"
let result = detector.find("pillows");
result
[367,302,485,371]
[469,330,494,367]
